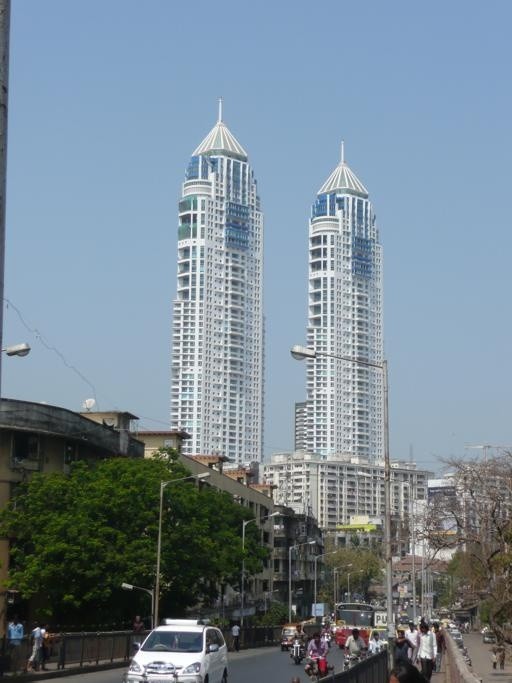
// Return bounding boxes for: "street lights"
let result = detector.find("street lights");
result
[354,470,417,628]
[347,570,364,602]
[240,512,281,648]
[122,581,154,632]
[288,539,316,624]
[1,342,31,357]
[313,550,337,622]
[289,345,398,683]
[333,563,353,620]
[154,470,211,627]
[264,588,279,617]
[395,539,433,622]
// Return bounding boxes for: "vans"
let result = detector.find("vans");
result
[124,624,229,683]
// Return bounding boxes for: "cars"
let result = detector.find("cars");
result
[392,599,421,626]
[448,621,496,664]
[280,615,337,650]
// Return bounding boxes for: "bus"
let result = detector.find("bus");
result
[333,602,388,650]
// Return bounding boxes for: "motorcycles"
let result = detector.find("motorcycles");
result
[301,653,333,680]
[340,649,366,670]
[289,635,307,664]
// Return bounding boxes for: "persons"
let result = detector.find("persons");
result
[231,623,241,651]
[291,617,446,682]
[491,651,497,668]
[9,615,50,672]
[498,650,506,670]
[133,614,145,643]
